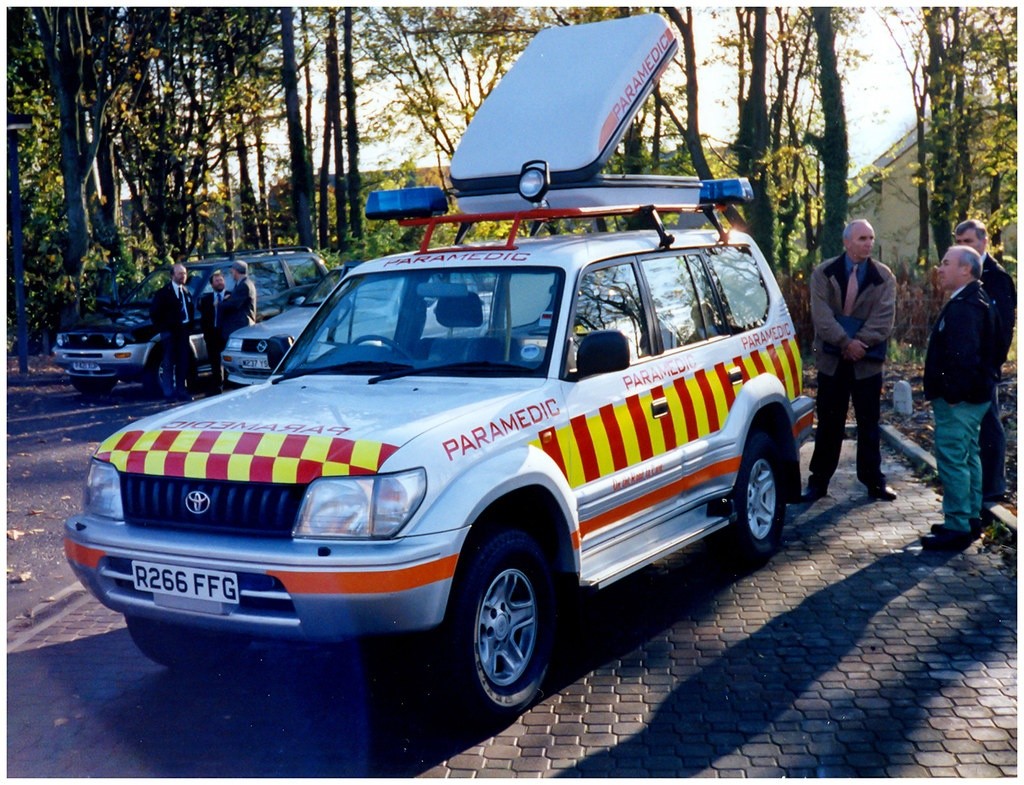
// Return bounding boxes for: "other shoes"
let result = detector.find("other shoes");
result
[868,487,897,501]
[165,392,177,403]
[931,518,983,539]
[982,492,1005,502]
[176,392,194,401]
[920,532,970,552]
[800,488,827,499]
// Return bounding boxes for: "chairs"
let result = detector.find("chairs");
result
[416,290,488,364]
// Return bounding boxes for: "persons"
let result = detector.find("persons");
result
[919,246,994,548]
[801,219,897,501]
[196,270,233,395]
[155,263,194,402]
[954,219,1017,502]
[220,260,256,339]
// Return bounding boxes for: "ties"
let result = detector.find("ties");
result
[843,264,858,316]
[216,294,222,327]
[177,287,187,320]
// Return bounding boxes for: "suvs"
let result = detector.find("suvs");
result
[50,245,338,402]
[219,267,492,388]
[63,12,819,717]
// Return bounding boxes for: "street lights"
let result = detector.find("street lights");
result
[7,113,33,372]
